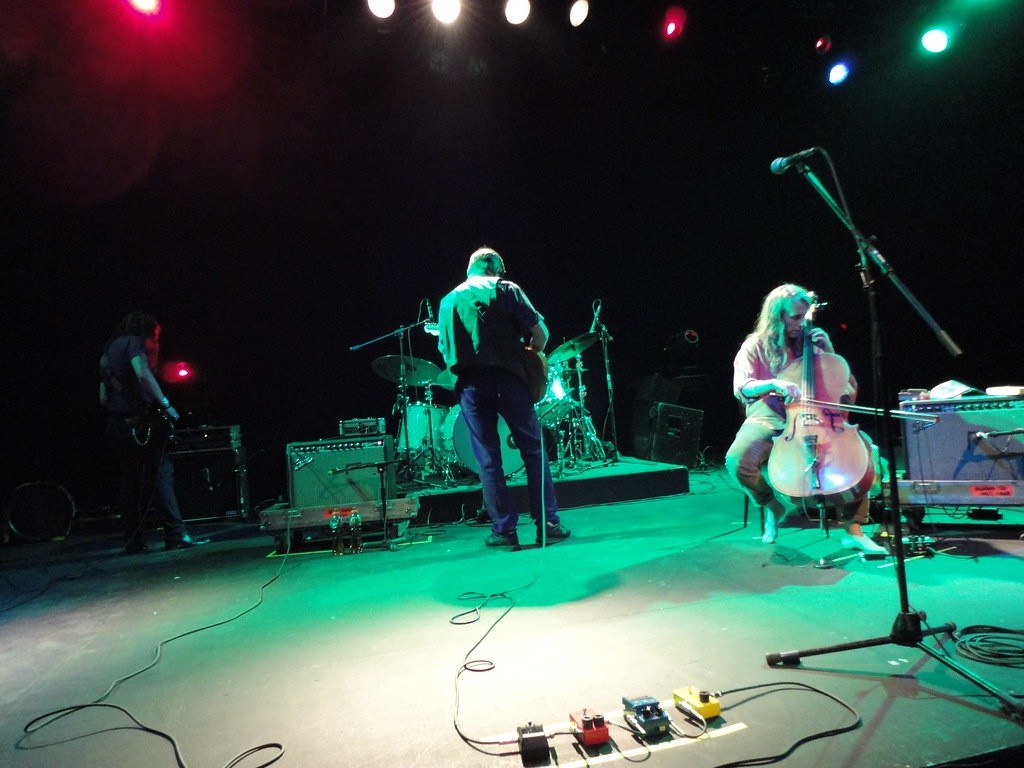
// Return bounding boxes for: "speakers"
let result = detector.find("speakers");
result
[168,447,250,522]
[285,435,396,510]
[897,393,1024,481]
[620,400,703,470]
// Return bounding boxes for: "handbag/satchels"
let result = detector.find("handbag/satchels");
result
[124,412,178,455]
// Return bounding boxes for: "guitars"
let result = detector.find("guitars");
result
[141,407,177,442]
[422,321,550,403]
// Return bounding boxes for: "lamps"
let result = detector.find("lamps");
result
[792,18,851,88]
[543,0,589,28]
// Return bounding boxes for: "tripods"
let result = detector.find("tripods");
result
[538,318,658,479]
[350,320,457,489]
[766,165,1024,717]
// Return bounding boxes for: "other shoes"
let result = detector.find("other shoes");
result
[844,534,888,556]
[761,505,785,543]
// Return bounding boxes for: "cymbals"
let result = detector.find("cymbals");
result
[547,330,599,366]
[372,353,442,387]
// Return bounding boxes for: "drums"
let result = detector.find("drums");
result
[440,403,527,481]
[396,400,449,464]
[533,361,573,426]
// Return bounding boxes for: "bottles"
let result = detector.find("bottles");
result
[348,506,364,554]
[329,507,346,556]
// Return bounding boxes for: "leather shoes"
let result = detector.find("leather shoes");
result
[126,537,177,555]
[165,531,212,552]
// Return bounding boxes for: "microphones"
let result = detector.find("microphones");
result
[328,467,340,474]
[770,147,820,175]
[975,431,982,441]
[426,299,435,322]
[590,303,602,333]
[200,468,212,491]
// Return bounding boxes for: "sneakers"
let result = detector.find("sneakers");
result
[485,530,521,551]
[535,521,570,544]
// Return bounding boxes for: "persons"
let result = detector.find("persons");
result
[99,311,212,554]
[725,283,890,555]
[438,247,570,552]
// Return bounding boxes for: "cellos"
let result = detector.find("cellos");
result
[764,290,878,570]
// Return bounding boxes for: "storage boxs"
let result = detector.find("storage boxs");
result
[174,424,241,452]
